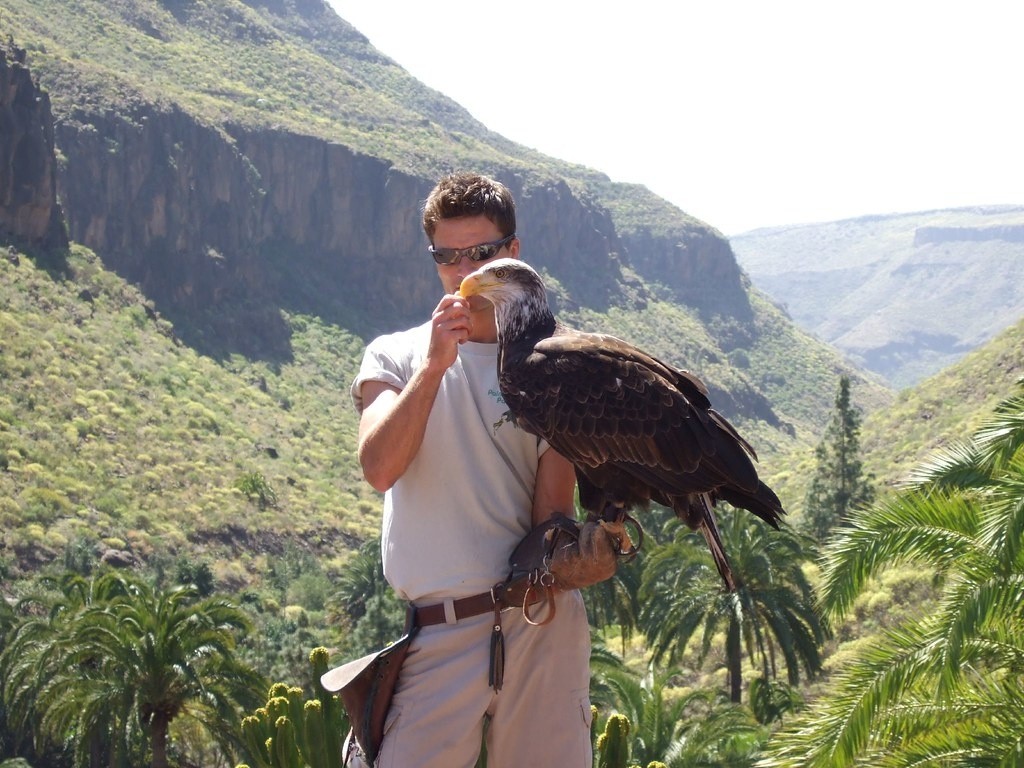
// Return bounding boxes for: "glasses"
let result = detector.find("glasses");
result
[428,234,517,265]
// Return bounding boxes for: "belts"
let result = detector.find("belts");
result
[415,580,509,629]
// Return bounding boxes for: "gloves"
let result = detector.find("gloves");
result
[549,522,617,591]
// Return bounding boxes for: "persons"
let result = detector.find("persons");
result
[356,173,617,768]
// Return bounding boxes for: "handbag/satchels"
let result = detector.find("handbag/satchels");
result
[320,625,422,767]
